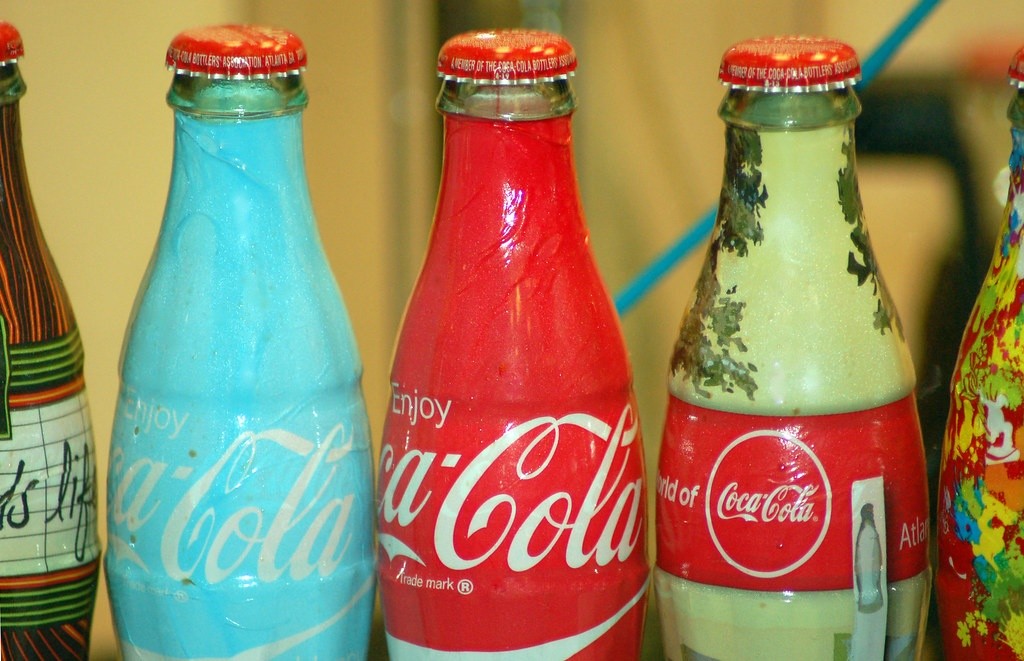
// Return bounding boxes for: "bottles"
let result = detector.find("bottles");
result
[937,46,1023,661]
[378,26,651,661]
[855,503,883,613]
[102,23,376,661]
[1,18,107,661]
[654,33,931,661]
